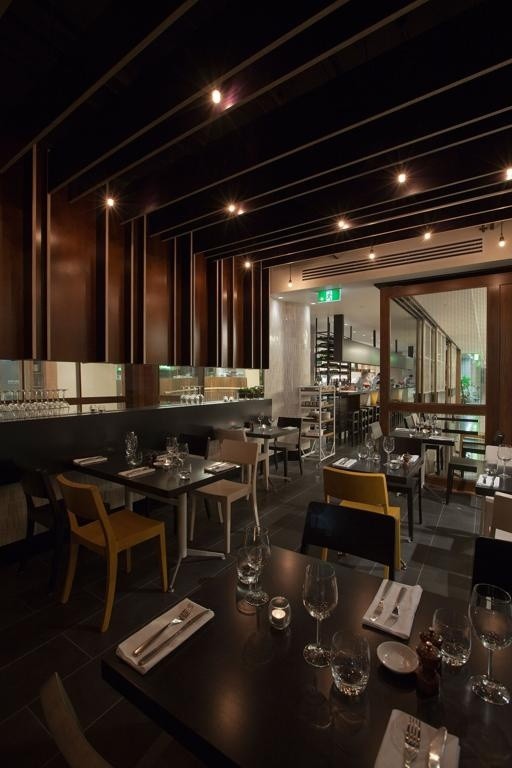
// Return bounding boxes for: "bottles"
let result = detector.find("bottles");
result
[224,394,234,402]
[403,452,412,471]
[268,597,292,630]
[412,627,444,698]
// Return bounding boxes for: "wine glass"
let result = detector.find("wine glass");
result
[245,526,272,605]
[415,413,442,437]
[180,385,204,406]
[355,433,396,467]
[303,561,339,668]
[167,437,192,467]
[257,411,278,433]
[468,584,512,705]
[0,386,71,419]
[497,443,511,479]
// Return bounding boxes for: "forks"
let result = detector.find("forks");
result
[401,718,422,767]
[133,604,194,654]
[384,584,407,629]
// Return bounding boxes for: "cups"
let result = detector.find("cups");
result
[237,389,265,401]
[331,630,371,696]
[388,454,401,473]
[180,464,192,479]
[91,404,106,413]
[235,548,263,584]
[432,608,471,667]
[409,425,417,435]
[483,458,498,474]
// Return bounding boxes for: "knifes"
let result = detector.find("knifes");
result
[491,475,496,486]
[370,578,394,623]
[138,610,209,665]
[339,457,350,464]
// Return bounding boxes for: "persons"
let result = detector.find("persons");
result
[373,373,380,388]
[356,369,371,391]
[399,374,414,388]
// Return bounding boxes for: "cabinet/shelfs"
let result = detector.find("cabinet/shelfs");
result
[313,331,352,387]
[298,385,335,465]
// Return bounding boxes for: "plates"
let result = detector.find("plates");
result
[376,641,419,674]
[338,388,357,393]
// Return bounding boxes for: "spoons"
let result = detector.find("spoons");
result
[427,727,446,767]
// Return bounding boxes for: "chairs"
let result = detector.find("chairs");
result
[343,405,380,445]
